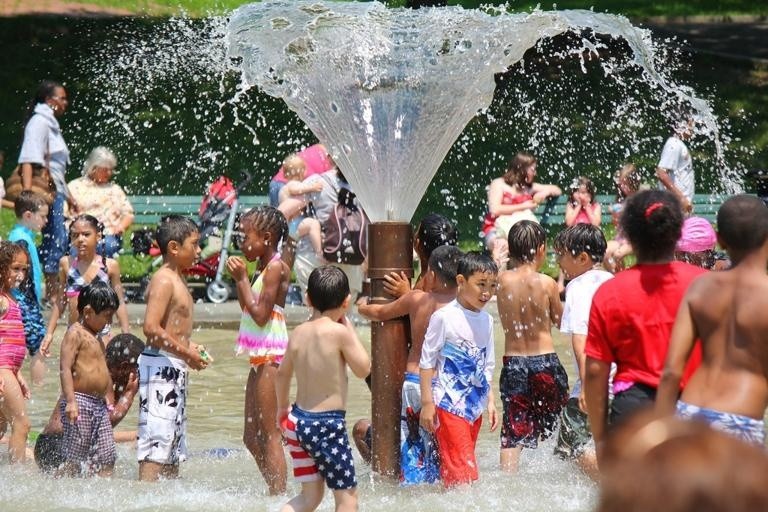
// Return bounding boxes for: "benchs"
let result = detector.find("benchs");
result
[485,194,758,258]
[127,196,271,255]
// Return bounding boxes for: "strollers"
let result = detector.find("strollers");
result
[121,169,249,305]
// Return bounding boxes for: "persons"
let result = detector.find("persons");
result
[657,105,694,218]
[585,186,768,511]
[0,76,210,481]
[223,137,649,511]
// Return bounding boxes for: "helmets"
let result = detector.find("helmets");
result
[678,218,717,255]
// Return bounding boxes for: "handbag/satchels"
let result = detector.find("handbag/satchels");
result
[495,209,539,239]
[8,166,58,208]
[133,227,157,255]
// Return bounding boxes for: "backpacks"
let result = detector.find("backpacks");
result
[321,172,367,267]
